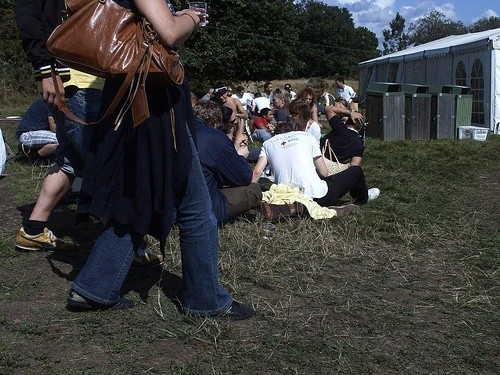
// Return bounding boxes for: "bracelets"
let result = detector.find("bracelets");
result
[181,13,197,29]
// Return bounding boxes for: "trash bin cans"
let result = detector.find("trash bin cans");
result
[365,81,406,141]
[403,84,432,140]
[430,85,456,139]
[454,85,473,140]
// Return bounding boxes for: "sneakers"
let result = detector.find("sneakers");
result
[219,299,254,320]
[67,290,134,312]
[130,250,164,271]
[368,187,380,202]
[16,227,77,253]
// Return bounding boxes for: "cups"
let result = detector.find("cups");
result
[260,222,276,239]
[188,1,207,26]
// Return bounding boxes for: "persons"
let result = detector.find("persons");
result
[190,82,321,162]
[250,123,380,215]
[14,0,106,252]
[16,96,60,165]
[288,78,358,140]
[320,106,366,168]
[65,0,255,321]
[192,101,263,226]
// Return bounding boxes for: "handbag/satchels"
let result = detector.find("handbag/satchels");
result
[261,202,304,221]
[46,0,184,125]
[322,139,350,177]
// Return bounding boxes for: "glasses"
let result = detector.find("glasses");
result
[228,118,235,125]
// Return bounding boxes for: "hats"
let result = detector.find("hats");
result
[213,84,228,95]
